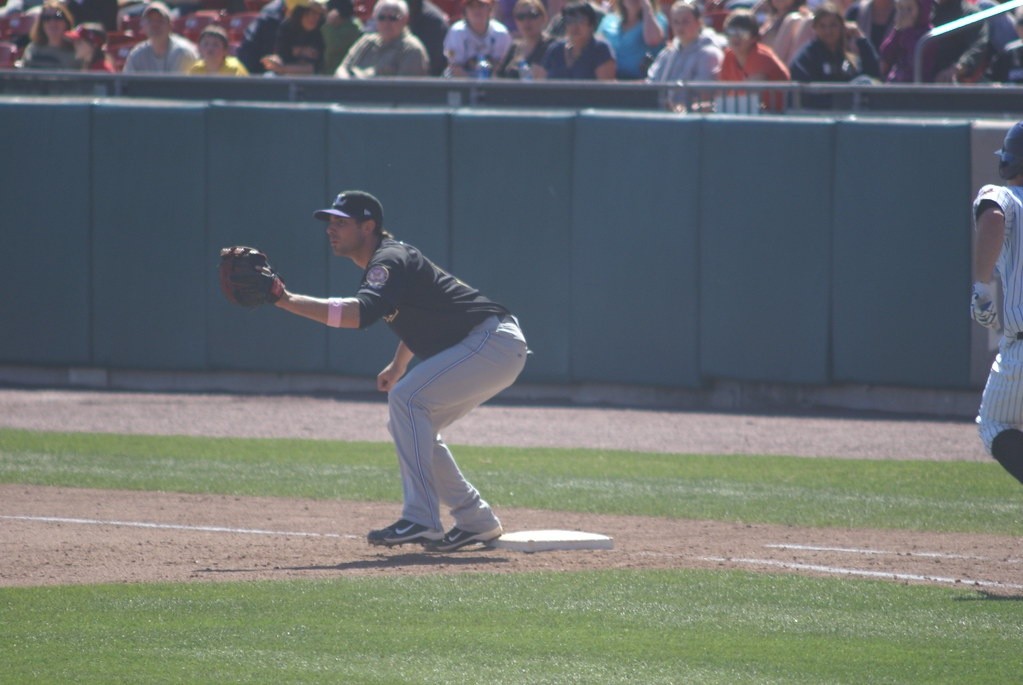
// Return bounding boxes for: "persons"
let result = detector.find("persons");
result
[971,118,1023,485]
[217,191,528,552]
[21,0,1023,124]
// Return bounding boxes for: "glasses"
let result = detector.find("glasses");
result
[42,11,68,22]
[514,10,542,21]
[375,12,404,22]
[728,26,754,40]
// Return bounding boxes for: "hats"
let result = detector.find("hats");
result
[313,189,384,227]
[63,24,103,46]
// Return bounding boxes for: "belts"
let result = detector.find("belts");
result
[1016,331,1023,341]
[497,313,519,325]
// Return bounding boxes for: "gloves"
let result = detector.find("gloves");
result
[968,281,1003,331]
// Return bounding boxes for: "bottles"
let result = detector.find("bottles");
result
[517,59,532,79]
[473,56,493,78]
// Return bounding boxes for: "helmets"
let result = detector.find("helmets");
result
[992,121,1023,180]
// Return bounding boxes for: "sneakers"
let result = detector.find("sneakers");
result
[429,520,505,552]
[367,518,449,547]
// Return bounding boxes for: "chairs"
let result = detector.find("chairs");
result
[0,0,732,74]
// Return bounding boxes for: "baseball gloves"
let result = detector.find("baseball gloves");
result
[216,246,287,308]
